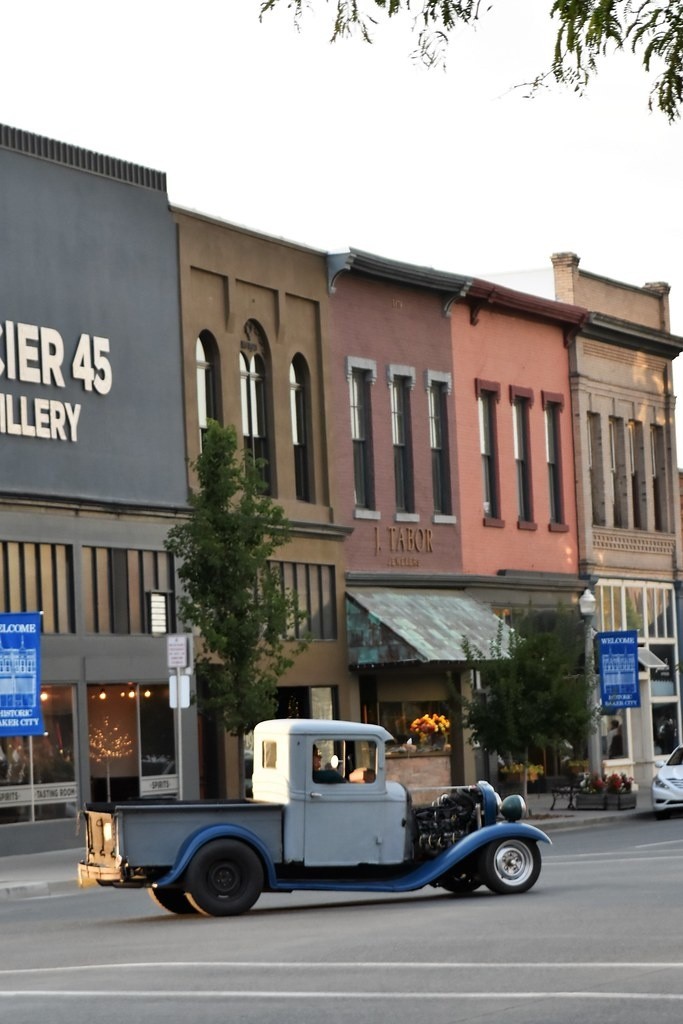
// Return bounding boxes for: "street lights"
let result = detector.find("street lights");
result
[578,586,605,785]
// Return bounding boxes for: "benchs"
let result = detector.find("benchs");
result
[548,775,585,810]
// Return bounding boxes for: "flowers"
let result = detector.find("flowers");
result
[580,771,634,793]
[502,763,543,777]
[410,713,450,750]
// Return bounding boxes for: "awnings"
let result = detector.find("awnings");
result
[345,587,527,666]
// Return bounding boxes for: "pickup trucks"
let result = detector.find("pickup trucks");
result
[76,717,553,919]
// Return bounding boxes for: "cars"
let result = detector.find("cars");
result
[650,744,683,820]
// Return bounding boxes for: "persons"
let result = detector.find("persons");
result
[606,721,624,761]
[312,745,347,783]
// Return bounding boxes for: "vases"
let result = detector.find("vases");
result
[576,792,636,812]
